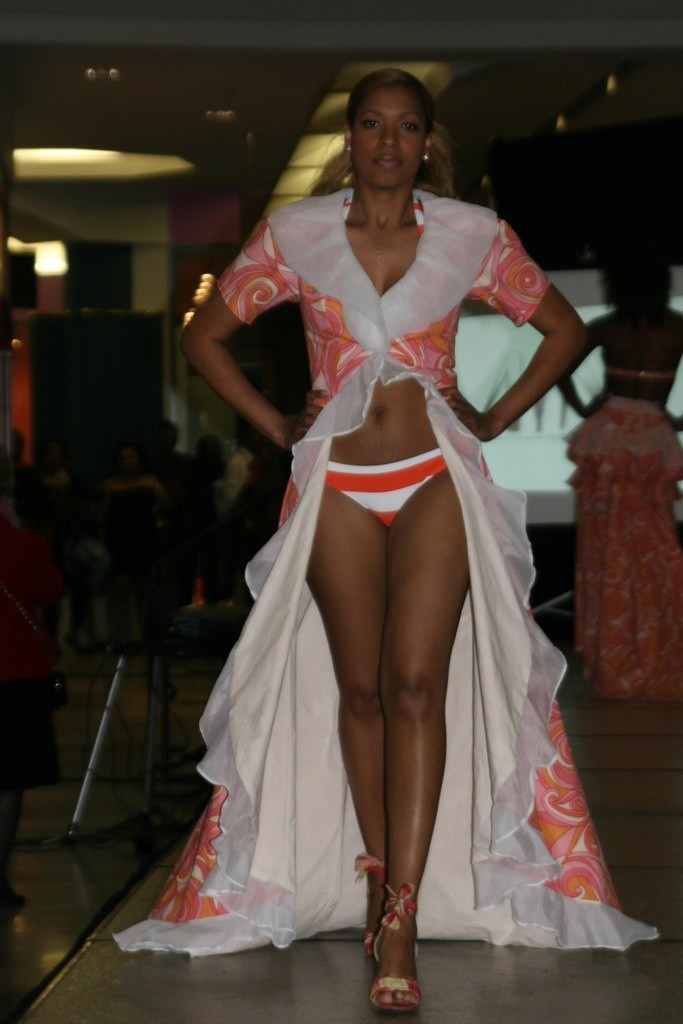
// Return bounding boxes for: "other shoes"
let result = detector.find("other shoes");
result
[63,631,94,653]
[0,879,24,907]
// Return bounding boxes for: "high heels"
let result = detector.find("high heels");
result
[370,883,422,1012]
[353,853,385,955]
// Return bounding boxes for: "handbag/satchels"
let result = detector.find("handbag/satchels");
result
[51,668,67,711]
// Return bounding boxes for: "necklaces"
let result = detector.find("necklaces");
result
[376,246,384,258]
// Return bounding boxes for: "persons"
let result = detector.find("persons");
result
[559,237,683,702]
[0,413,300,908]
[113,69,661,1014]
